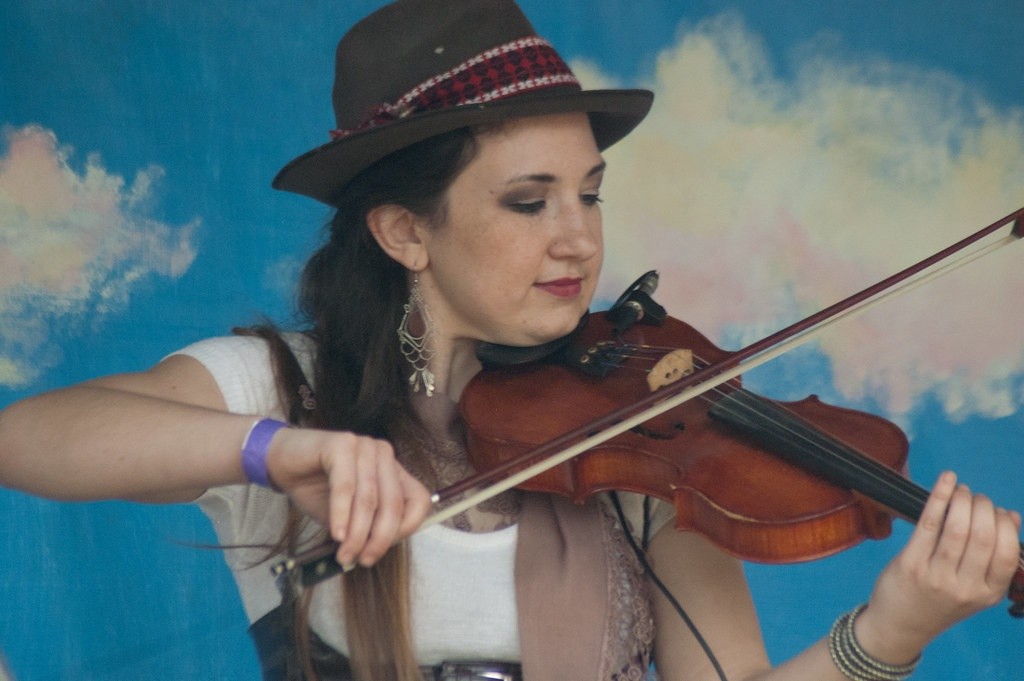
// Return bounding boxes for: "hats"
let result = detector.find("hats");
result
[272,0,654,206]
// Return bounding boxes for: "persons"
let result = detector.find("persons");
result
[0,0,1022,681]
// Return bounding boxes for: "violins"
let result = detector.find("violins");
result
[457,269,1024,615]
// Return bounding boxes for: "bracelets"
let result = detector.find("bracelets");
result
[827,603,921,680]
[241,417,287,486]
